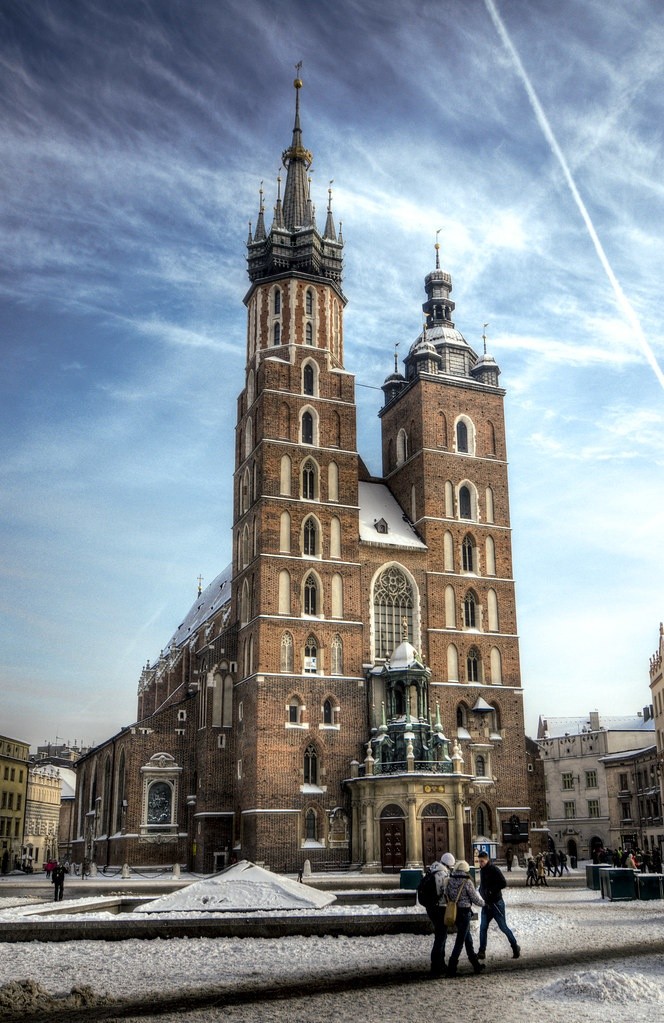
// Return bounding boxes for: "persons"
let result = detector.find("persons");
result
[82,858,89,880]
[592,847,663,873]
[46,862,53,878]
[505,847,513,871]
[448,860,486,974]
[526,849,569,887]
[474,852,521,959]
[52,862,69,901]
[427,852,456,972]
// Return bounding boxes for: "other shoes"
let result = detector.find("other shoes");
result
[526,882,549,887]
[59,898,62,901]
[507,869,511,871]
[55,900,57,902]
[548,872,562,877]
[430,961,448,973]
[511,943,521,958]
[476,952,486,959]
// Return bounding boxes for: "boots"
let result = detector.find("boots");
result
[468,954,485,975]
[447,957,462,977]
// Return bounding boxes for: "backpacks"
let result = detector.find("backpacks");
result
[443,879,467,925]
[417,869,444,907]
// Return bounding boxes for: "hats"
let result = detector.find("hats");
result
[441,852,455,866]
